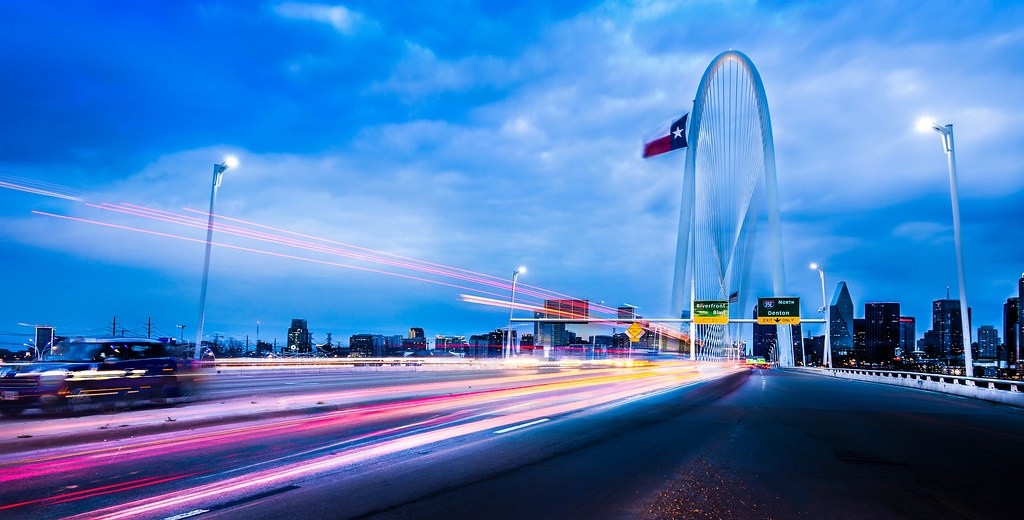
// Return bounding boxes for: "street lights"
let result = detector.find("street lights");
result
[505,266,528,359]
[193,153,240,362]
[916,113,975,385]
[809,261,833,368]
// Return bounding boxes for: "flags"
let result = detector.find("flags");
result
[642,110,688,158]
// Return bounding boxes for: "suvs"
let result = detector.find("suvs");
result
[0,335,180,419]
